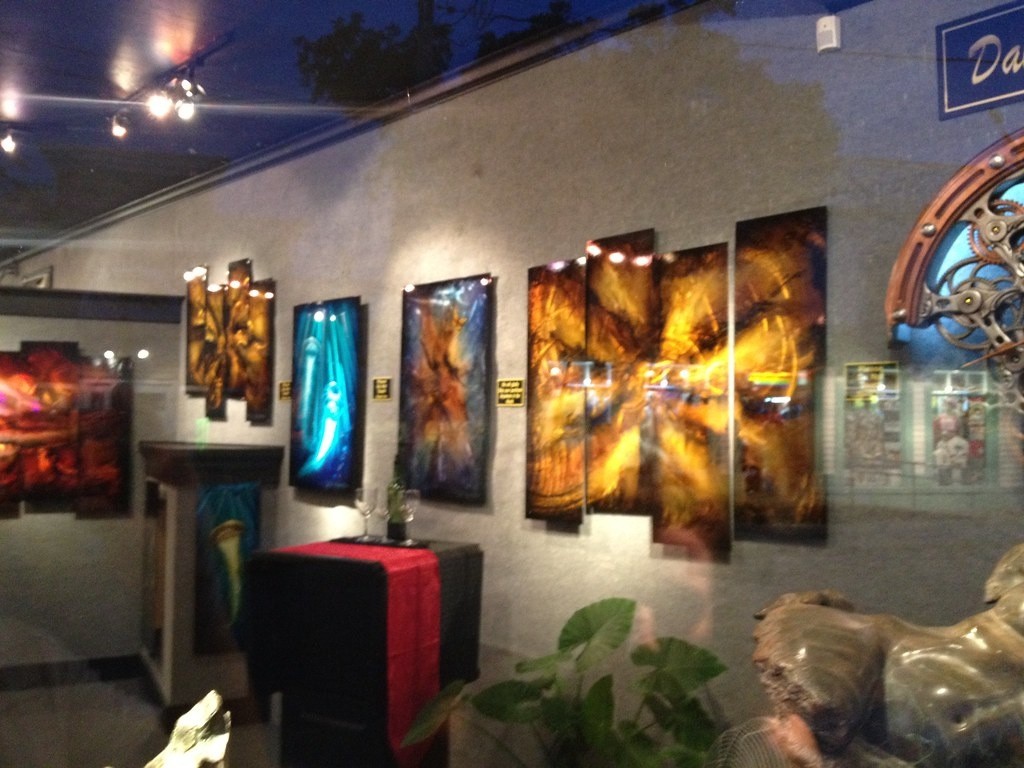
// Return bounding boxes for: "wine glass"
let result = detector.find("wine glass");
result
[354,486,378,542]
[400,489,420,547]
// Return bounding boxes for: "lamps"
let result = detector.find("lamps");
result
[112,115,125,137]
[148,66,206,120]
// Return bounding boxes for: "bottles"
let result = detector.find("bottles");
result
[386,454,406,540]
